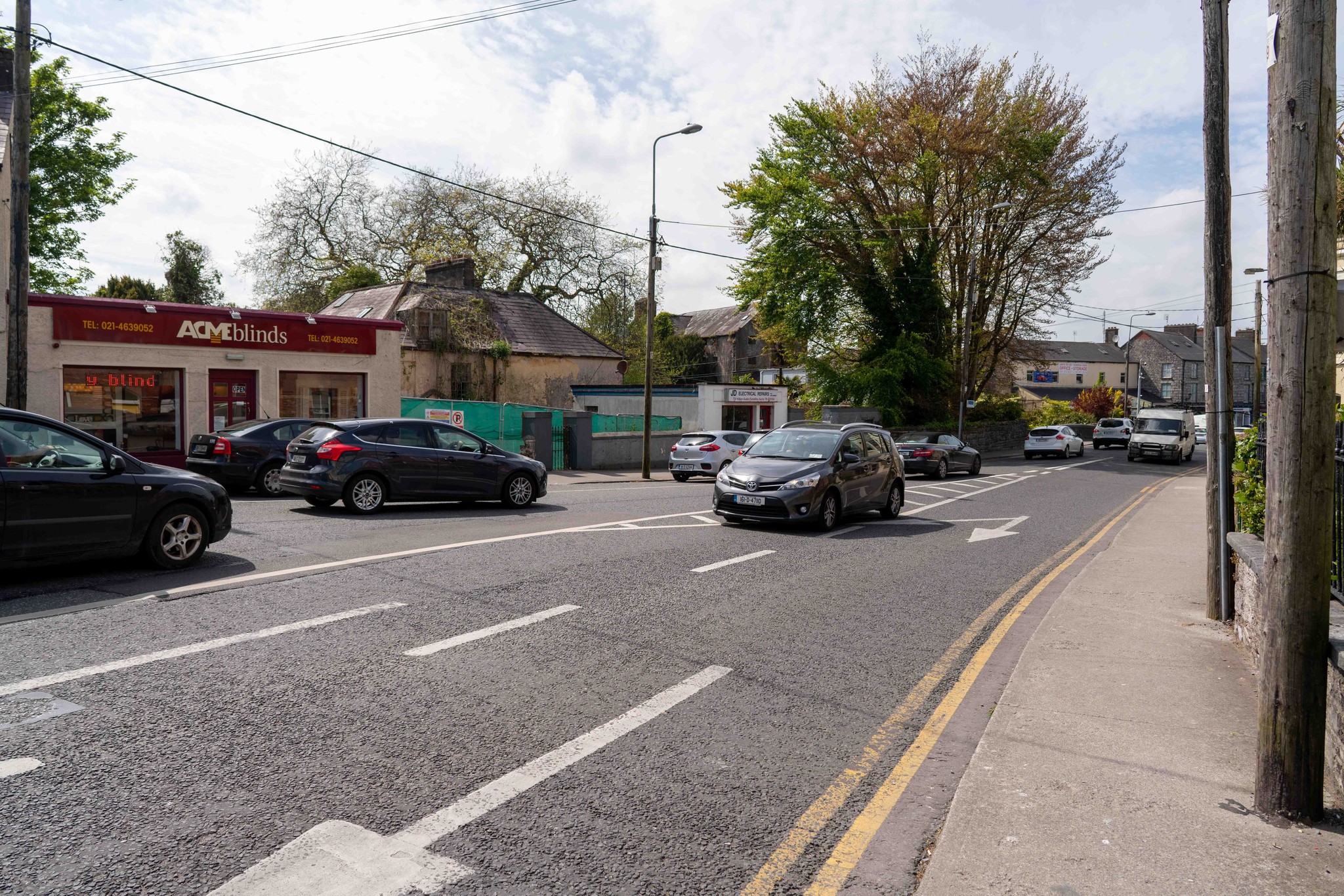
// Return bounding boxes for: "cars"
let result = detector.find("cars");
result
[1195,427,1206,445]
[894,431,982,480]
[186,416,333,499]
[1023,425,1084,460]
[0,405,234,570]
[278,417,549,517]
[1234,424,1254,436]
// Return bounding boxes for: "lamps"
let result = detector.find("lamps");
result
[305,313,317,324]
[143,300,157,313]
[229,308,242,320]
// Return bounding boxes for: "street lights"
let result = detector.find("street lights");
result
[957,200,1012,439]
[641,123,705,477]
[1124,311,1156,418]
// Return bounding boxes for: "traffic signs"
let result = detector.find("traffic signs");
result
[967,400,977,408]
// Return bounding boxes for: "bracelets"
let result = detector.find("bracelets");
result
[39,447,48,452]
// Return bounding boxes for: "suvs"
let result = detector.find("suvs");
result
[668,430,752,482]
[1092,417,1135,449]
[738,427,797,458]
[711,418,906,532]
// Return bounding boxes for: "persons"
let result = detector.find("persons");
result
[5,446,54,465]
[439,428,463,451]
[786,442,804,456]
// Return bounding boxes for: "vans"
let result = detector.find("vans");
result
[1125,408,1195,466]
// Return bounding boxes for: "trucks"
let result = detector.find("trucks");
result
[1194,413,1206,432]
